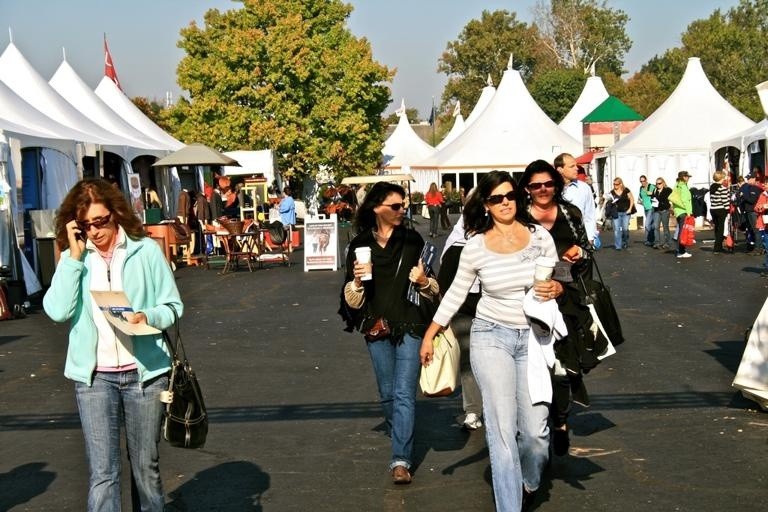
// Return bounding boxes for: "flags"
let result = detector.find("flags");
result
[104,38,121,91]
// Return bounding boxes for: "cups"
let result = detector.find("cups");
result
[73,211,112,230]
[640,181,645,182]
[485,191,516,203]
[379,202,405,210]
[527,181,557,189]
[657,183,661,185]
[613,183,620,185]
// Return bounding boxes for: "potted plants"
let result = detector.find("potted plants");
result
[126,172,145,224]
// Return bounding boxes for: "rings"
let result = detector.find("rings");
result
[547,293,552,298]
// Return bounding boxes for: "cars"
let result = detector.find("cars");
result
[354,246,372,281]
[532,256,555,300]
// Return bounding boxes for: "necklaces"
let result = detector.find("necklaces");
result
[375,233,390,239]
[533,205,553,212]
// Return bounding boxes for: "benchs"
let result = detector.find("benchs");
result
[104,38,121,91]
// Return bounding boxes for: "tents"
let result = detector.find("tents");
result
[711,120,768,179]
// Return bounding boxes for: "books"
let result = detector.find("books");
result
[627,215,644,232]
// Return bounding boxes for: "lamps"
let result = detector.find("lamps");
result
[220,235,255,274]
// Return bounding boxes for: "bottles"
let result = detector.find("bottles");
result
[676,171,692,181]
[744,172,755,178]
[441,185,446,188]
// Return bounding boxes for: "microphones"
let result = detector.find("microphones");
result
[190,257,201,266]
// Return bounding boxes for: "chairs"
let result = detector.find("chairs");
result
[448,225,452,230]
[433,233,438,238]
[554,424,569,456]
[662,243,670,248]
[430,232,432,237]
[464,413,482,429]
[643,241,655,246]
[653,243,660,248]
[676,252,692,258]
[393,466,412,484]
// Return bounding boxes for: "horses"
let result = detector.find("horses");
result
[73,228,88,245]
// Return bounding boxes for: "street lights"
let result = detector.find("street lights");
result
[421,205,430,219]
[161,361,209,448]
[628,200,637,214]
[580,279,624,346]
[418,325,460,397]
[723,214,731,236]
[362,316,391,342]
[726,236,734,248]
[668,188,686,209]
[584,303,617,361]
[680,217,695,246]
[445,199,454,208]
[734,206,748,231]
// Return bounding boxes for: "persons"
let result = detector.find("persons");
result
[177,189,190,226]
[274,187,296,226]
[41,176,185,512]
[436,189,484,430]
[609,177,634,251]
[638,171,696,256]
[418,169,565,512]
[221,182,253,219]
[148,190,163,209]
[710,169,768,254]
[211,189,223,249]
[425,183,465,238]
[515,159,594,458]
[337,180,440,486]
[318,183,367,221]
[196,193,208,243]
[555,154,599,245]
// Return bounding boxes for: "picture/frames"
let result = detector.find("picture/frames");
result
[145,223,190,270]
[256,224,293,269]
[411,202,463,213]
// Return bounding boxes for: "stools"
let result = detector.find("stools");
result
[429,205,439,206]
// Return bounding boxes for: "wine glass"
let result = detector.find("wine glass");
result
[547,293,552,298]
